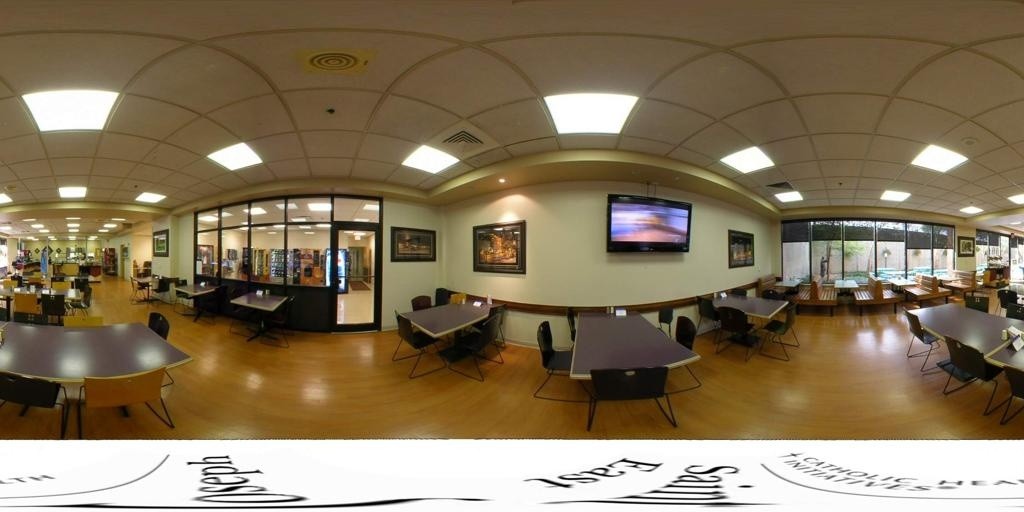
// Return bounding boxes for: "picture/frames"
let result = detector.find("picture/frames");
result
[390,226,436,263]
[151,228,169,255]
[727,229,755,269]
[957,235,976,258]
[472,220,527,275]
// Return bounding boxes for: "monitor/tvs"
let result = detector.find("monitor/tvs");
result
[606,193,692,253]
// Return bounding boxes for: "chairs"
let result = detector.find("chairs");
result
[694,271,1024,425]
[392,292,702,433]
[0,261,300,439]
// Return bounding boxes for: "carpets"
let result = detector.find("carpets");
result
[350,281,371,292]
